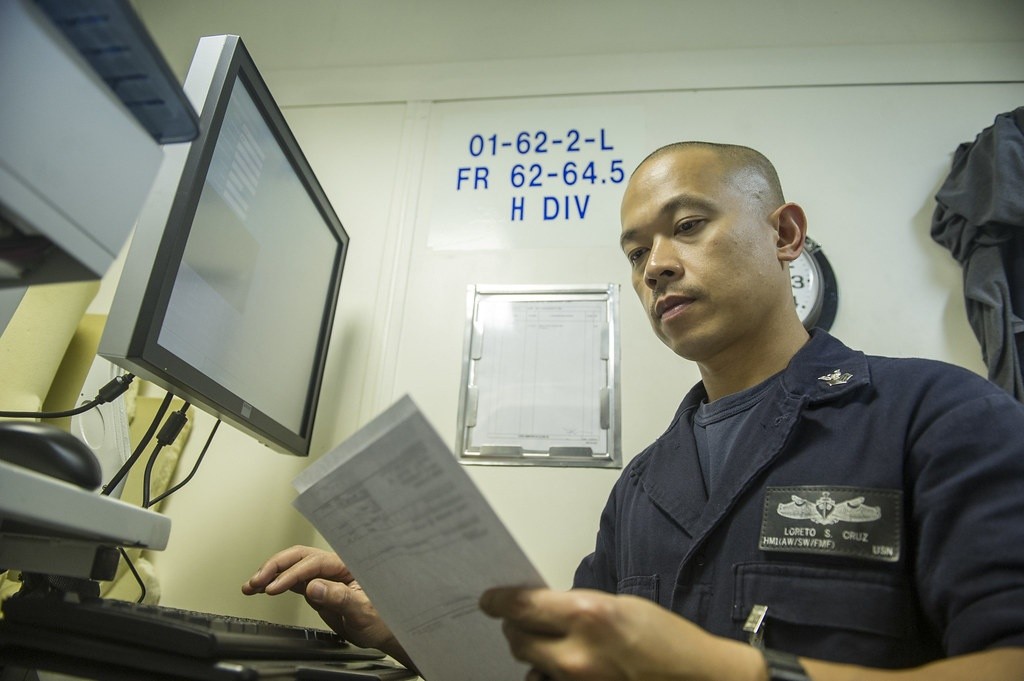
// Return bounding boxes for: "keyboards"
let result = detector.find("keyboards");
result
[0,590,386,659]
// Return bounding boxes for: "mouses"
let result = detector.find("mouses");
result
[0,419,102,493]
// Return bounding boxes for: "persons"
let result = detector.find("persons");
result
[242,140,1024,681]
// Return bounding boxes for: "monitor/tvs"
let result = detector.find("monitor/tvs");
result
[72,34,350,500]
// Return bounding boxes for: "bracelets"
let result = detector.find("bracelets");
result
[759,648,813,681]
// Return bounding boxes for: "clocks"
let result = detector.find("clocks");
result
[789,237,837,334]
